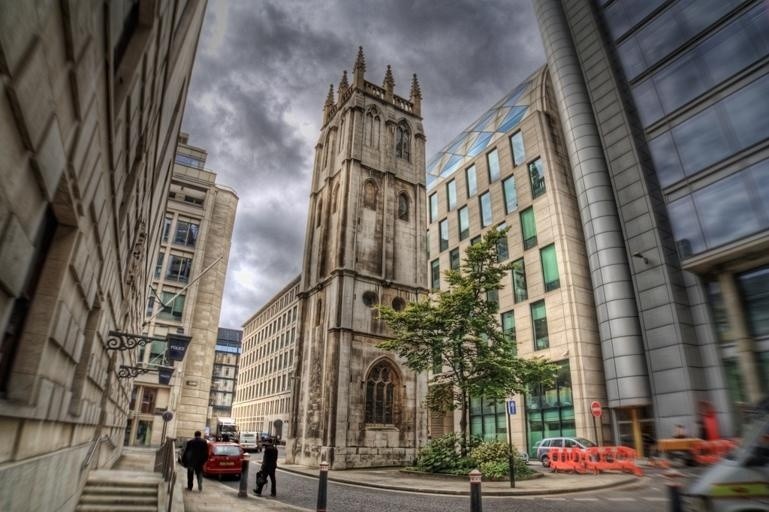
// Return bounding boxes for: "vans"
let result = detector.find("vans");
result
[240,432,262,452]
[538,438,599,467]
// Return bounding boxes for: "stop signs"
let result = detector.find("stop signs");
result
[591,401,602,417]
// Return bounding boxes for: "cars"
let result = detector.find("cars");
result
[201,441,247,481]
[261,433,275,446]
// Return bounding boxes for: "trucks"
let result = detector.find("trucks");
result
[216,418,238,442]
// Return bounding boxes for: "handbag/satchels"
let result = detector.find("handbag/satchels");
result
[256,472,267,487]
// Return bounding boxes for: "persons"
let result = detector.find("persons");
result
[253,437,277,495]
[671,423,687,439]
[182,430,208,491]
[221,429,230,441]
